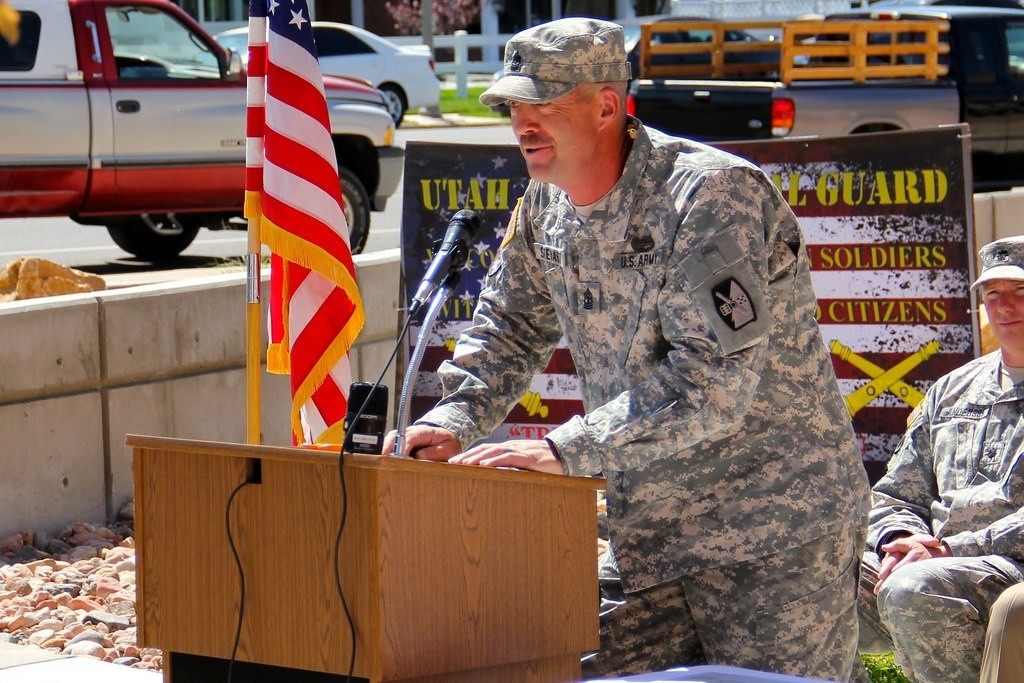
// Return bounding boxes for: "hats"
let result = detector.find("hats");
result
[969,235,1024,291]
[477,16,633,108]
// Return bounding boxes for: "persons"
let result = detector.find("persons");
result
[865,235,1024,683]
[378,18,871,683]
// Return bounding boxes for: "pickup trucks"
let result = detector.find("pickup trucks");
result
[1,1,406,257]
[626,4,1023,193]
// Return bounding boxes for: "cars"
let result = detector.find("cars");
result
[192,21,442,128]
[606,14,782,88]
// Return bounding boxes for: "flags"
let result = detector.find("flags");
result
[245,0,366,446]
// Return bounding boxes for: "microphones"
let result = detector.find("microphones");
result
[408,208,481,316]
